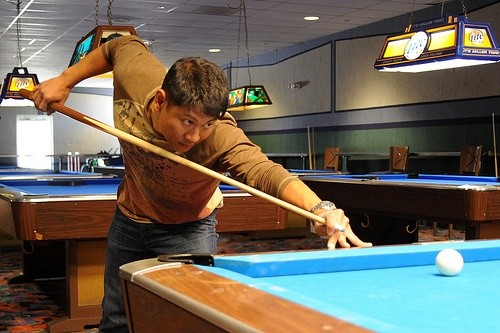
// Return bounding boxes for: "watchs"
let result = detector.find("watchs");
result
[310,200,337,214]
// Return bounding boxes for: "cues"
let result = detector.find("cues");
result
[19,86,345,232]
[308,127,318,170]
[491,113,499,177]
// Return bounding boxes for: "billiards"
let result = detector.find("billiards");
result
[435,249,464,276]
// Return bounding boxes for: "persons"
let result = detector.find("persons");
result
[32,35,372,333]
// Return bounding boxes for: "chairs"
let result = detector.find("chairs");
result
[324,148,340,171]
[433,144,482,239]
[369,146,410,174]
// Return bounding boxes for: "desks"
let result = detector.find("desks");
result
[297,174,500,246]
[94,165,125,175]
[0,178,289,333]
[0,166,102,178]
[117,239,500,333]
[219,169,342,190]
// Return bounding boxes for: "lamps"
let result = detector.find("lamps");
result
[68,0,138,79]
[227,0,273,112]
[373,0,500,74]
[0,0,40,106]
